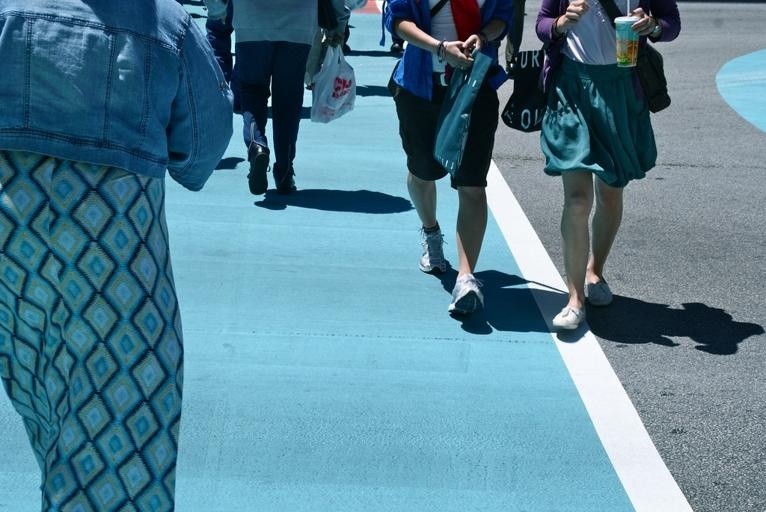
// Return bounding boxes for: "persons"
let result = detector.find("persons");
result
[379,0,514,317]
[505,0,526,79]
[205,0,240,113]
[390,36,405,53]
[201,0,351,196]
[535,0,682,332]
[0,0,234,512]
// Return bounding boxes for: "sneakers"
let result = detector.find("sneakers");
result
[447,271,486,318]
[418,228,448,276]
[549,304,588,332]
[584,279,615,307]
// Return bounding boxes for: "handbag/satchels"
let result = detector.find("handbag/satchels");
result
[634,41,675,117]
[501,48,561,136]
[318,0,339,31]
[433,48,494,181]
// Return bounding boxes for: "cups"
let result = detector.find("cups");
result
[614,16,640,68]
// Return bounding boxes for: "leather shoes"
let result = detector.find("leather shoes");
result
[243,142,270,197]
[273,163,299,195]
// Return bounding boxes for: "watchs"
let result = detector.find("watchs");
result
[649,20,663,40]
[436,40,448,64]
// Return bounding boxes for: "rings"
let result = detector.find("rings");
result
[460,65,464,72]
